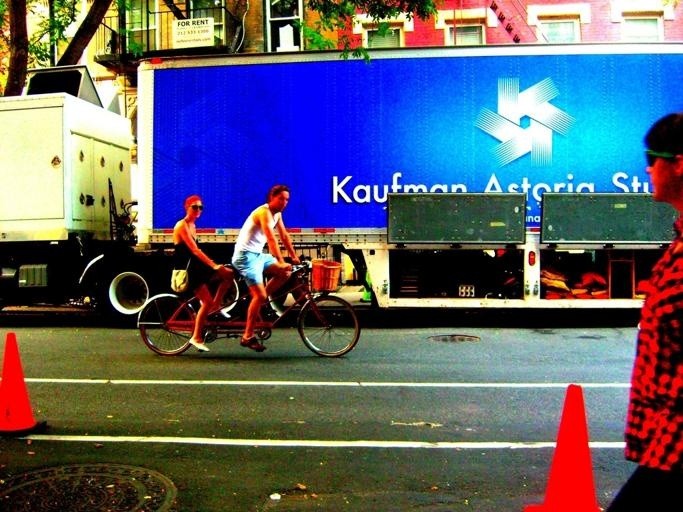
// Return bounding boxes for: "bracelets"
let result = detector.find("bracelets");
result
[213,264,222,269]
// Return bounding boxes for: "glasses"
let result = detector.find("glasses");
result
[645,150,676,167]
[189,205,203,210]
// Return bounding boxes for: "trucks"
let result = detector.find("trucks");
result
[0,40,682,324]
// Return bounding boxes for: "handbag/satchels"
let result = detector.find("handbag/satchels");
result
[171,269,189,293]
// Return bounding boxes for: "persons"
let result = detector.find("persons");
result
[232,184,300,352]
[604,113,683,512]
[173,195,234,352]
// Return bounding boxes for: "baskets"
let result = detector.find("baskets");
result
[312,259,342,291]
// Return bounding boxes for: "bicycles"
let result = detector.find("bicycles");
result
[138,253,363,359]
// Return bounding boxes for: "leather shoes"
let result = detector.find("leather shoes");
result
[240,336,266,350]
[189,337,210,351]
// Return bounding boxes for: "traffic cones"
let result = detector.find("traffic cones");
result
[523,382,605,511]
[0,331,45,437]
